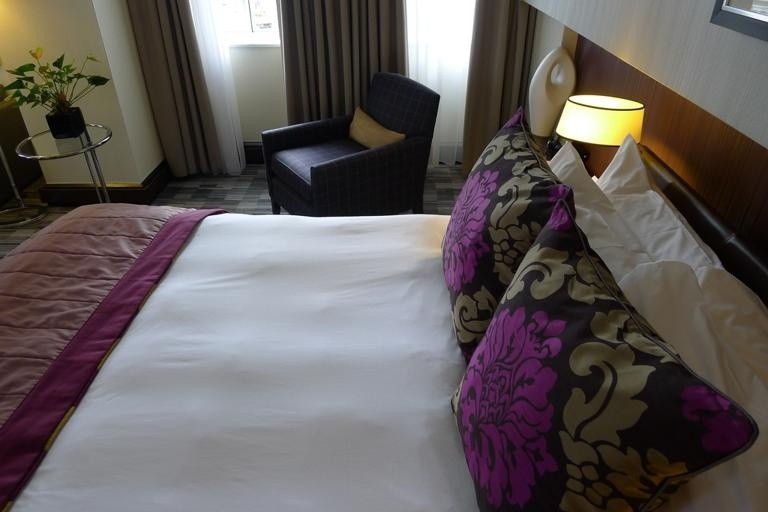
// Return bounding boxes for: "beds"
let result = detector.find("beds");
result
[0,146,768,512]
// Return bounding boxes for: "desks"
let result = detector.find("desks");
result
[15,125,117,205]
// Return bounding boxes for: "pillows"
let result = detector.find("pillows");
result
[347,104,407,151]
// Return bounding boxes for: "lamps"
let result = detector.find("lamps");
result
[553,92,646,172]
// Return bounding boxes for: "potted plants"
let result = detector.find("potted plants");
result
[0,38,112,138]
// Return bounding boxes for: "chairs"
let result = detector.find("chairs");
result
[258,70,442,215]
